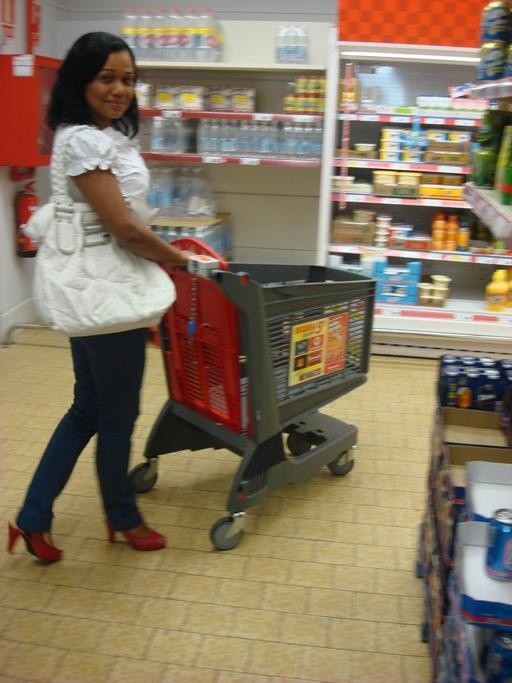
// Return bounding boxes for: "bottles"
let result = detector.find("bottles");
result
[429,211,470,252]
[131,115,321,161]
[118,4,223,64]
[273,22,309,64]
[147,164,231,258]
[484,269,509,315]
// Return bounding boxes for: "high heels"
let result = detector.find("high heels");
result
[4,516,63,562]
[103,511,167,552]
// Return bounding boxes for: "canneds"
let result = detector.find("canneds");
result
[469,0,512,189]
[439,353,512,411]
[486,630,512,683]
[484,507,512,580]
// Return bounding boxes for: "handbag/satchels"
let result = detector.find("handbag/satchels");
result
[17,121,178,337]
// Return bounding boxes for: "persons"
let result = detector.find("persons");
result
[1,28,203,566]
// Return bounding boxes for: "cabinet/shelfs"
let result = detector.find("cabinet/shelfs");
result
[128,59,324,169]
[315,42,511,360]
[462,78,511,240]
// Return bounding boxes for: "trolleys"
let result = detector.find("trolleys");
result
[130,240,377,551]
